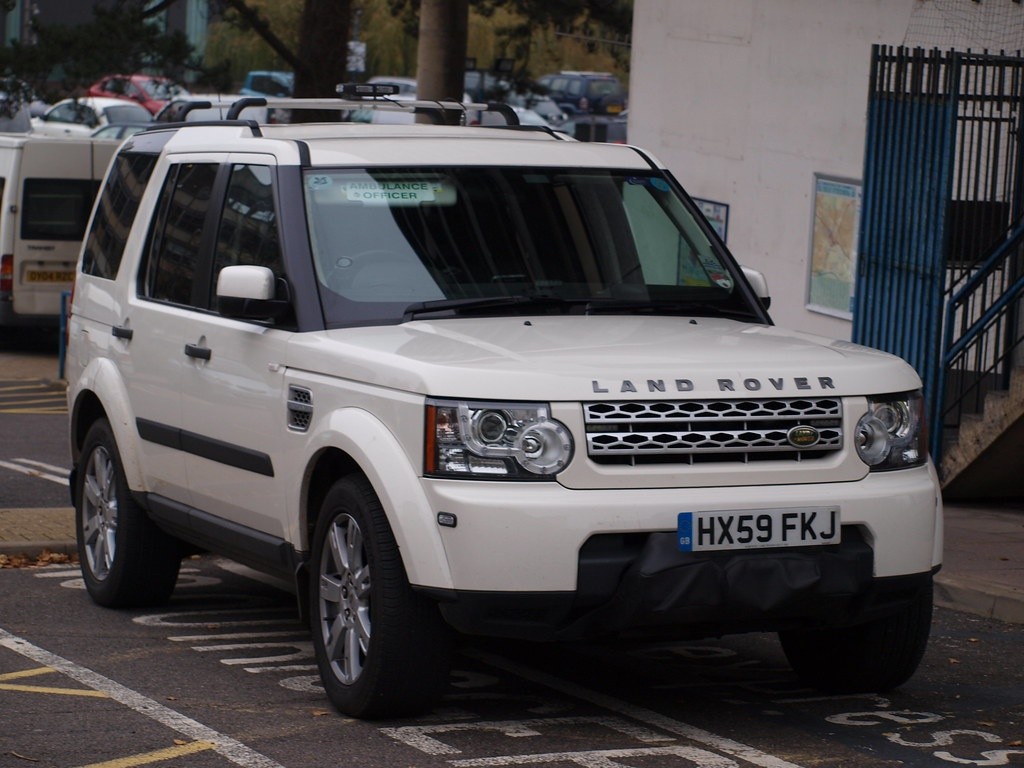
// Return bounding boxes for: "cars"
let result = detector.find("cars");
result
[0,41,630,354]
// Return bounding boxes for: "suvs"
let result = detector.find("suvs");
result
[63,81,946,724]
